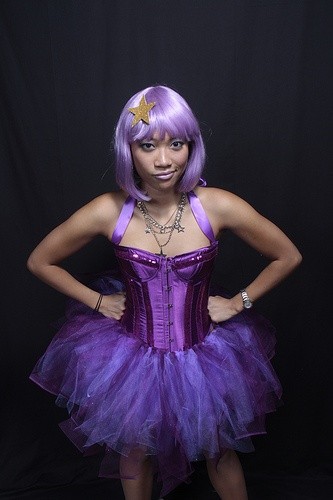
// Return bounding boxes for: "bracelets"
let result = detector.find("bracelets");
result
[93,293,103,312]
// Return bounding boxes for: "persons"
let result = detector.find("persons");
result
[26,85,301,500]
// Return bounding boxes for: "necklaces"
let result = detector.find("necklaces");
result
[137,190,186,257]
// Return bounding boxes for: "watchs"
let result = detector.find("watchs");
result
[239,288,253,310]
